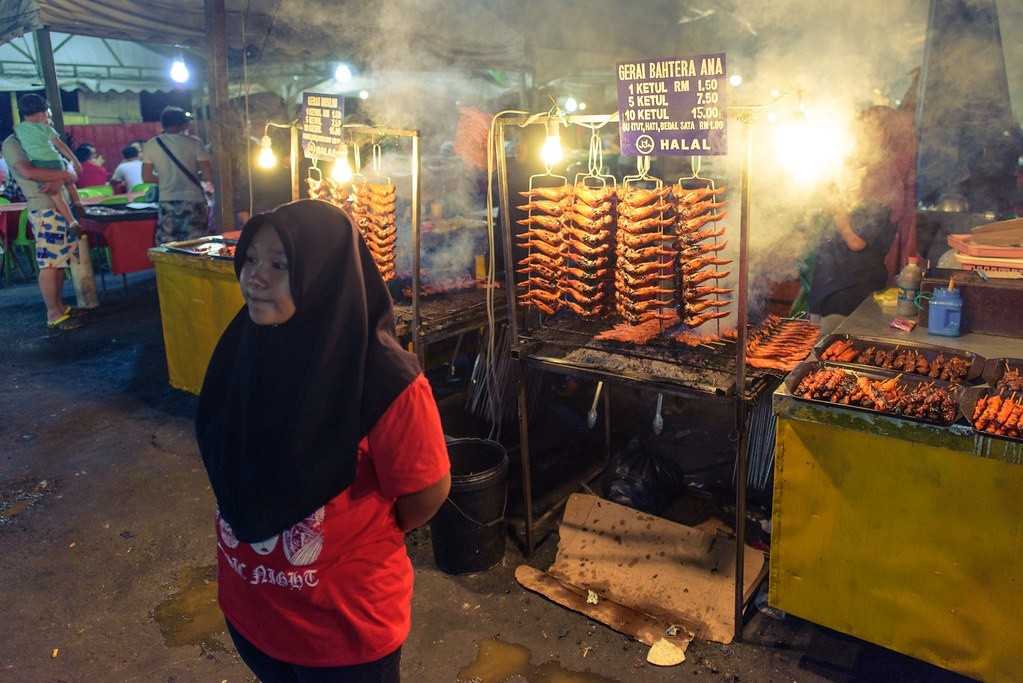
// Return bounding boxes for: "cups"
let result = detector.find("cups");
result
[927,288,963,337]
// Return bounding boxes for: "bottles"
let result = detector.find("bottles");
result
[897,257,922,317]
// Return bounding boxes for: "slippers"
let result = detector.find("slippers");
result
[47,315,84,332]
[64,306,88,318]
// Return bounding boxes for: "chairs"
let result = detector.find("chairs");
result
[0,183,158,280]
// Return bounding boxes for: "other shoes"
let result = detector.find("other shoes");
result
[76,201,86,216]
[68,221,81,236]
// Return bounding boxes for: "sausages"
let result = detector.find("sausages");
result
[821,340,860,363]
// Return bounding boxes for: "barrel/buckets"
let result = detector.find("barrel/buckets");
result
[429,438,509,576]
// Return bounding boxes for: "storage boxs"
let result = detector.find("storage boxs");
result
[954,250,1023,274]
[919,267,1023,339]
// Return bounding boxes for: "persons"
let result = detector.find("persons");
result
[0,93,892,323]
[195,199,450,682]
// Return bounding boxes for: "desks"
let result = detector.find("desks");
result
[76,192,159,299]
[0,202,73,284]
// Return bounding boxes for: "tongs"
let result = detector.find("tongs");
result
[653,393,663,435]
[586,381,603,429]
